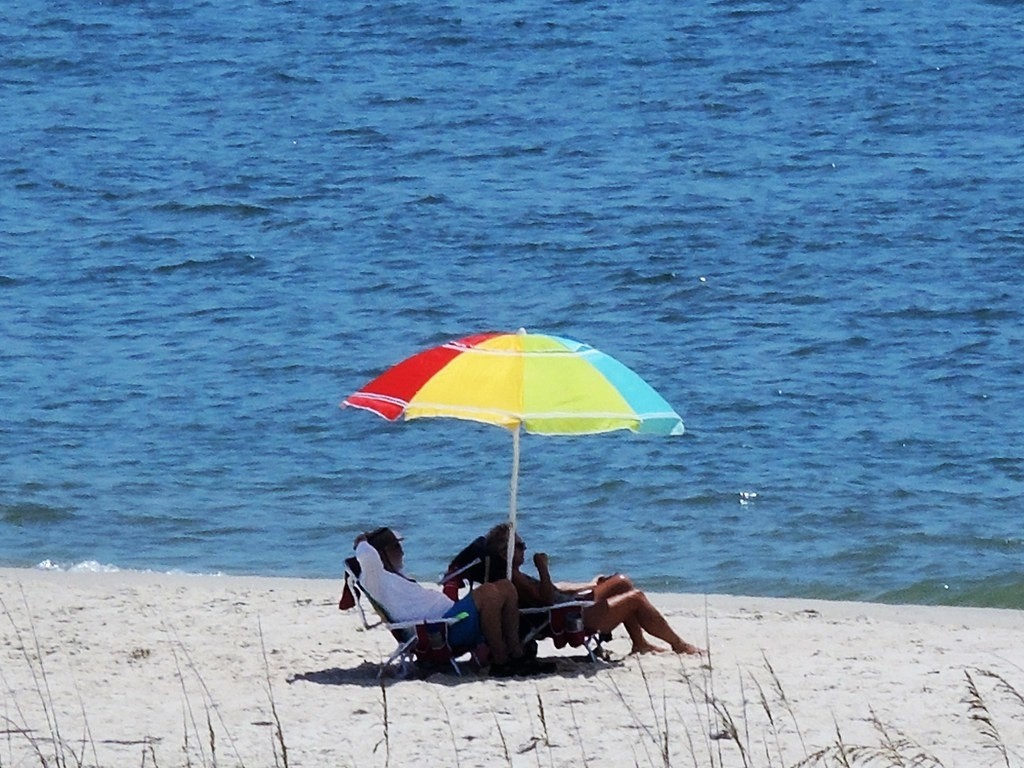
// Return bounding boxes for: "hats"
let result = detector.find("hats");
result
[366,527,404,550]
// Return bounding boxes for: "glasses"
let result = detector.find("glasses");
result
[519,542,527,551]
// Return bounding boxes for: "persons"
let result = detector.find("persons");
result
[481,523,709,656]
[353,526,558,678]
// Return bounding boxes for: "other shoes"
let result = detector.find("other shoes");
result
[513,660,555,673]
[488,662,528,677]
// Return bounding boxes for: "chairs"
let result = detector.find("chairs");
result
[339,557,481,684]
[438,535,604,663]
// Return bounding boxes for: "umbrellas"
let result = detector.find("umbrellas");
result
[341,327,686,582]
